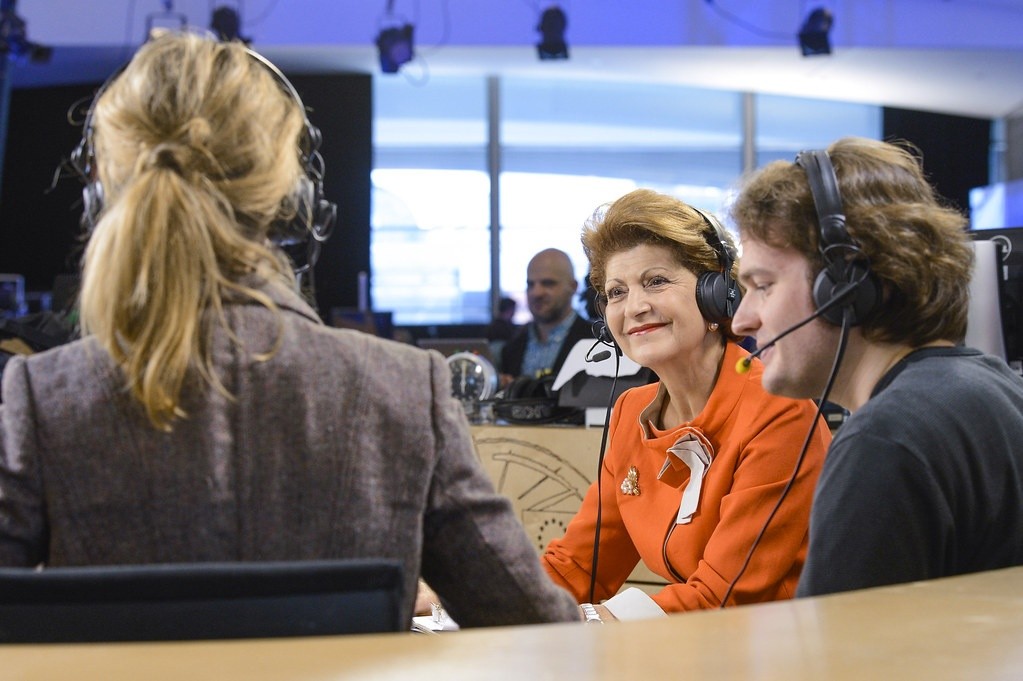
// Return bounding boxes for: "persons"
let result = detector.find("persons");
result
[414,188,833,623]
[489,297,517,342]
[501,247,596,388]
[733,137,1023,597]
[0,32,579,629]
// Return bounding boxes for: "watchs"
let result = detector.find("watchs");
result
[580,603,604,625]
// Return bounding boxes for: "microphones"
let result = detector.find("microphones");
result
[735,312,819,374]
[585,340,611,362]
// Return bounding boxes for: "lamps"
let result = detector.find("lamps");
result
[371,0,415,74]
[211,6,251,42]
[534,9,569,59]
[797,9,837,56]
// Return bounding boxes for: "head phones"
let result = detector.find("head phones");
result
[793,148,883,327]
[72,47,337,276]
[589,206,742,356]
